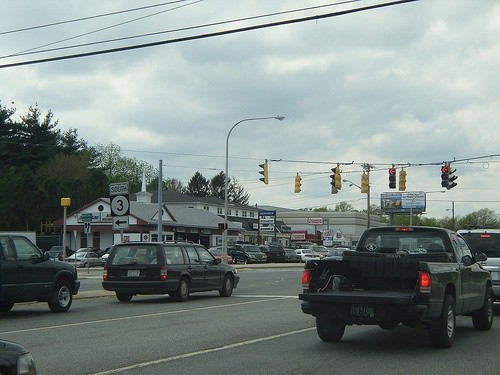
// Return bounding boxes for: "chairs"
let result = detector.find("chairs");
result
[427,243,445,253]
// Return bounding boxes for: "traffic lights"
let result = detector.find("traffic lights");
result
[441,166,449,187]
[330,167,341,190]
[258,163,268,185]
[389,168,396,189]
[447,168,458,190]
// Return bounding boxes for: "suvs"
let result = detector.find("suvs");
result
[0,233,80,315]
[455,229,500,310]
[102,239,240,303]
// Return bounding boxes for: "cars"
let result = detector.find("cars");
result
[44,246,113,268]
[0,339,37,375]
[204,242,356,264]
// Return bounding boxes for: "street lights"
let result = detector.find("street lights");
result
[410,190,446,226]
[446,201,455,231]
[342,179,370,229]
[223,116,285,263]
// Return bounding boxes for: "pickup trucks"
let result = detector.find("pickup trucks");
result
[298,224,494,349]
